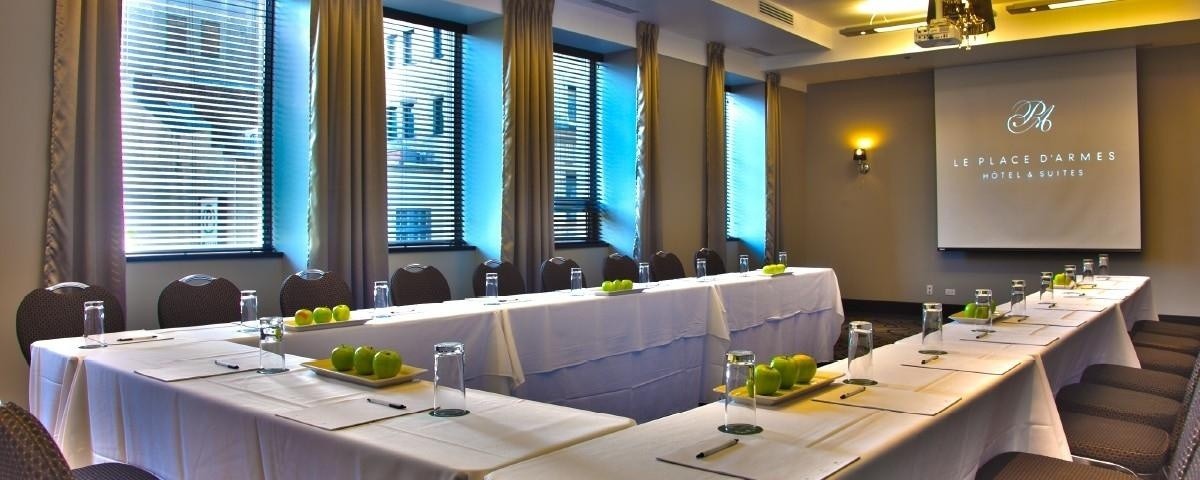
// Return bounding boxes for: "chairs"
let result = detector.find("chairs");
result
[1,248,1200,480]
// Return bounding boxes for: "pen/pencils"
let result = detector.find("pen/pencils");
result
[117,336,157,341]
[215,360,239,369]
[499,299,518,302]
[697,439,738,458]
[367,399,406,409]
[840,388,865,398]
[976,333,987,338]
[922,355,938,364]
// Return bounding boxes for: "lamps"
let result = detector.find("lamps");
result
[853,148,870,176]
[1006,1,1117,15]
[839,13,928,38]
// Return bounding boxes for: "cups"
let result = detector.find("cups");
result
[259,317,285,373]
[696,258,707,282]
[373,281,390,318]
[1064,265,1076,293]
[434,342,466,417]
[740,255,750,277]
[639,263,649,289]
[921,302,943,345]
[779,252,787,270]
[240,290,258,329]
[1098,254,1110,278]
[486,273,499,304]
[570,268,583,295]
[84,300,104,347]
[1083,258,1095,283]
[975,288,992,330]
[1011,280,1026,315]
[1039,272,1053,302]
[848,321,873,383]
[724,352,757,432]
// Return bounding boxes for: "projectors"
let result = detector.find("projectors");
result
[914,24,962,48]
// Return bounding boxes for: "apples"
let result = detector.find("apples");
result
[602,281,614,291]
[622,280,633,289]
[372,350,401,378]
[313,307,331,323]
[770,265,778,273]
[1055,273,1070,285]
[612,280,624,291]
[965,303,976,317]
[332,305,350,321]
[295,309,313,326]
[331,343,354,371]
[990,300,996,314]
[354,346,377,375]
[975,306,987,318]
[777,264,785,272]
[763,266,773,273]
[746,365,781,397]
[793,355,817,383]
[770,356,798,390]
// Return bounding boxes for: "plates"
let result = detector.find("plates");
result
[756,271,795,276]
[1050,281,1075,290]
[591,288,645,296]
[281,316,373,332]
[948,309,1011,324]
[300,357,429,388]
[713,370,846,406]
